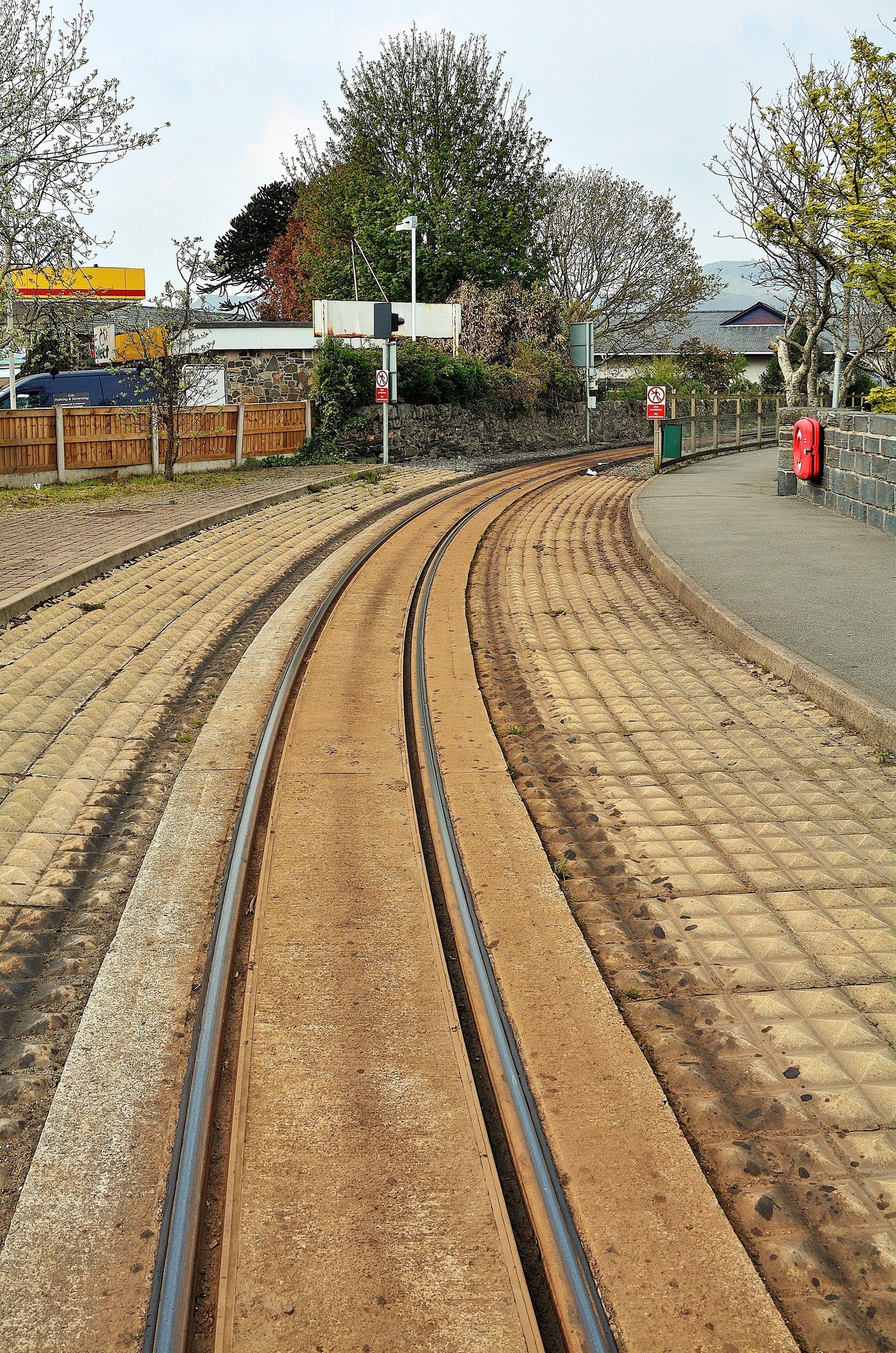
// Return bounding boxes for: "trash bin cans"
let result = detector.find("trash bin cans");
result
[663,423,682,458]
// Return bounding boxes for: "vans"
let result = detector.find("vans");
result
[0,365,164,410]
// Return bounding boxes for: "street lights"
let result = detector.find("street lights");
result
[396,215,417,345]
